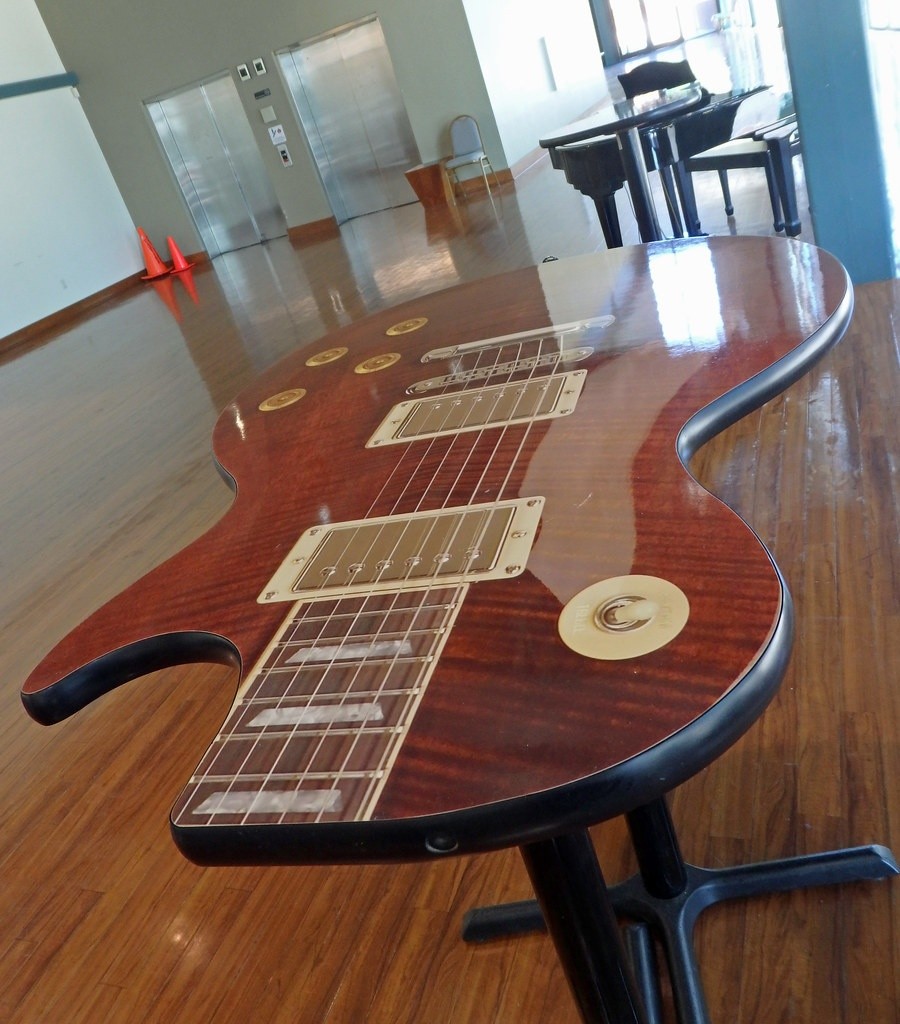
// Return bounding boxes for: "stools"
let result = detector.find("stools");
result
[690,114,800,243]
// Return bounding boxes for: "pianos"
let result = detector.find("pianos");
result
[538,59,779,249]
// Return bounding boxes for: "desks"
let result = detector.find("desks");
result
[18,235,900,1024]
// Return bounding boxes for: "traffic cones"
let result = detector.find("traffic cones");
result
[166,235,195,274]
[170,266,201,305]
[143,275,185,324]
[137,226,174,280]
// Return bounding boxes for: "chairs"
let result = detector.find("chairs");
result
[445,115,501,206]
